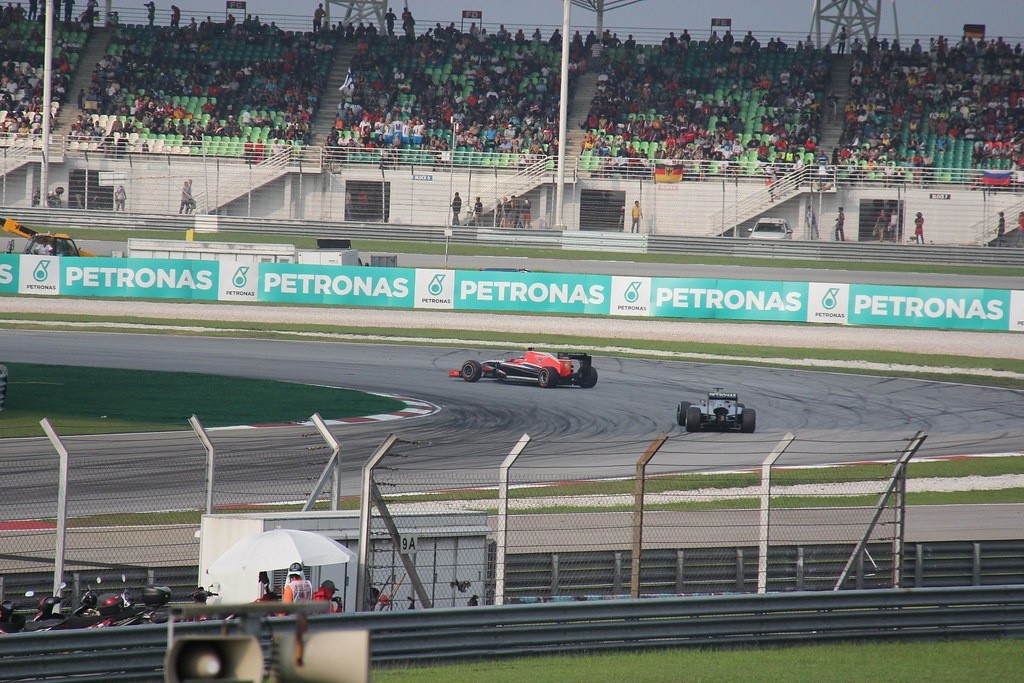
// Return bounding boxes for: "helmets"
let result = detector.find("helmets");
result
[1019,210,1024,215]
[288,563,303,577]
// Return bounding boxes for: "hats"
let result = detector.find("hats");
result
[322,580,340,591]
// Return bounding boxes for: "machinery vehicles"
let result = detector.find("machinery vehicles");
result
[0,218,94,256]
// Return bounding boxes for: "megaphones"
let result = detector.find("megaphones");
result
[164,633,264,683]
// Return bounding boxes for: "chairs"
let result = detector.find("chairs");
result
[0,17,1024,185]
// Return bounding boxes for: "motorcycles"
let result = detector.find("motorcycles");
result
[0,590,69,658]
[26,583,124,652]
[72,574,219,626]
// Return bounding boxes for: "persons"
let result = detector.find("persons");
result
[180,182,188,214]
[915,212,924,244]
[888,210,898,243]
[765,179,777,202]
[115,186,127,211]
[279,563,313,616]
[994,211,1024,247]
[0,0,1024,192]
[874,209,888,242]
[617,201,643,233]
[834,207,845,241]
[34,238,53,256]
[806,206,821,240]
[311,580,339,613]
[186,178,196,214]
[495,195,530,228]
[452,192,484,226]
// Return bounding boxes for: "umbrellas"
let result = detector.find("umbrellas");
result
[206,525,359,602]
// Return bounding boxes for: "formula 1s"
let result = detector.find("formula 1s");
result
[677,387,756,433]
[449,347,598,389]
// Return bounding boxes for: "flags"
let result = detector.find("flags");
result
[656,163,683,182]
[984,170,1012,185]
